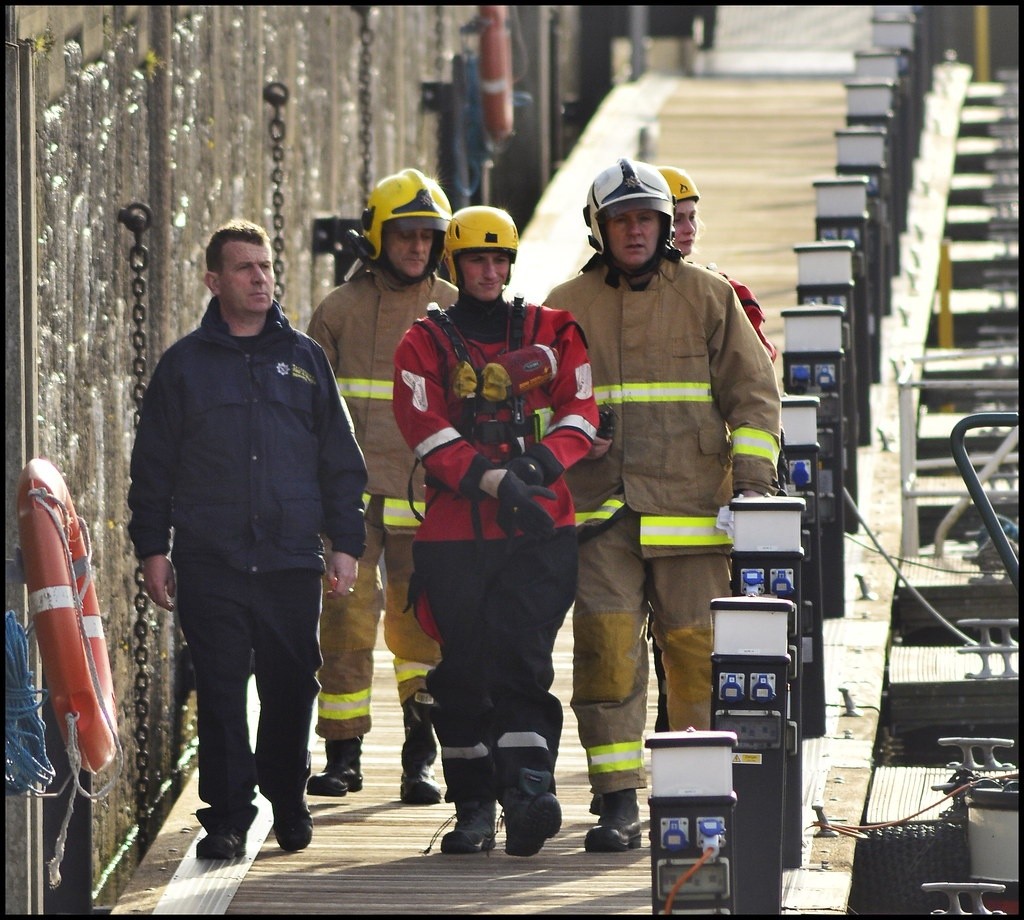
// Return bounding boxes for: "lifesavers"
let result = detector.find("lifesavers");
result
[478,6,515,141]
[16,457,119,775]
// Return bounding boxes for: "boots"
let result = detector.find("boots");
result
[423,799,497,853]
[307,735,363,797]
[584,788,641,853]
[401,739,441,805]
[499,768,562,857]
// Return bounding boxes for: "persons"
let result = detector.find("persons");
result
[128,219,369,858]
[647,165,777,733]
[542,159,782,852]
[307,169,460,805]
[393,205,599,857]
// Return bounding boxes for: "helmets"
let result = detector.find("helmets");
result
[444,206,518,286]
[362,168,452,263]
[585,158,700,254]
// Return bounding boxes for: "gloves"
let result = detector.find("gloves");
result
[494,470,556,541]
[505,444,565,487]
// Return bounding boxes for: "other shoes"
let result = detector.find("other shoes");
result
[272,795,313,852]
[196,824,248,860]
